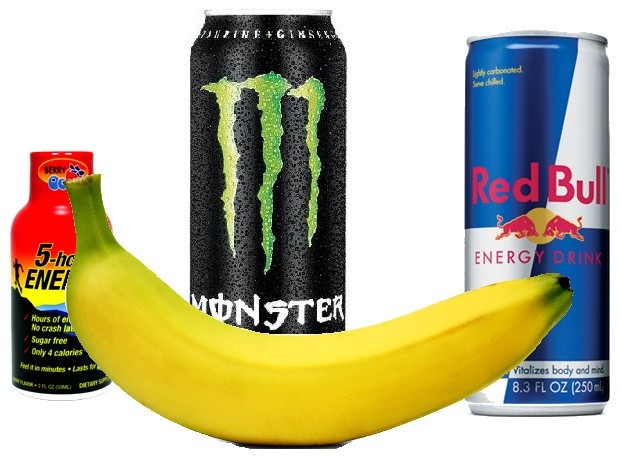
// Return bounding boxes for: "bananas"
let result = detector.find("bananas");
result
[62,173,575,444]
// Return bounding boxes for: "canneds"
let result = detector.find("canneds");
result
[184,6,350,452]
[458,28,612,418]
